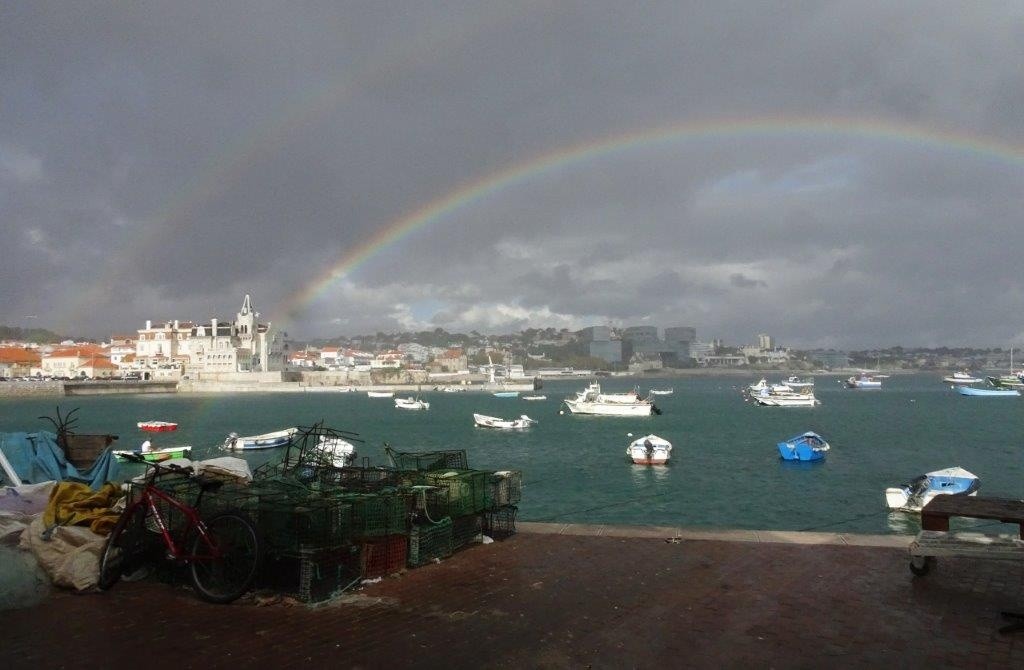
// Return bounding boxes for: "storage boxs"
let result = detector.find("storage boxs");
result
[163,448,192,460]
[141,452,168,463]
[59,433,119,468]
[114,454,137,464]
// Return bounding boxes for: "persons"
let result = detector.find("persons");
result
[142,437,154,452]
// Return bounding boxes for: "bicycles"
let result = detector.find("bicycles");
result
[97,449,261,605]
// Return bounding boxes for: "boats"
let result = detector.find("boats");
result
[338,387,358,392]
[220,426,300,452]
[367,392,397,398]
[136,420,179,433]
[942,371,984,384]
[649,388,676,397]
[950,385,1021,395]
[777,431,831,463]
[563,387,665,416]
[301,434,358,470]
[493,392,520,398]
[626,434,673,465]
[843,365,891,388]
[110,444,194,463]
[883,465,981,515]
[573,380,638,403]
[433,385,466,394]
[523,393,548,402]
[393,396,430,410]
[746,374,823,408]
[472,412,538,431]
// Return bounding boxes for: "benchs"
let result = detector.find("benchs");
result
[920,490,1024,540]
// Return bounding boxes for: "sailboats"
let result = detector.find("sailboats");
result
[987,345,1024,389]
